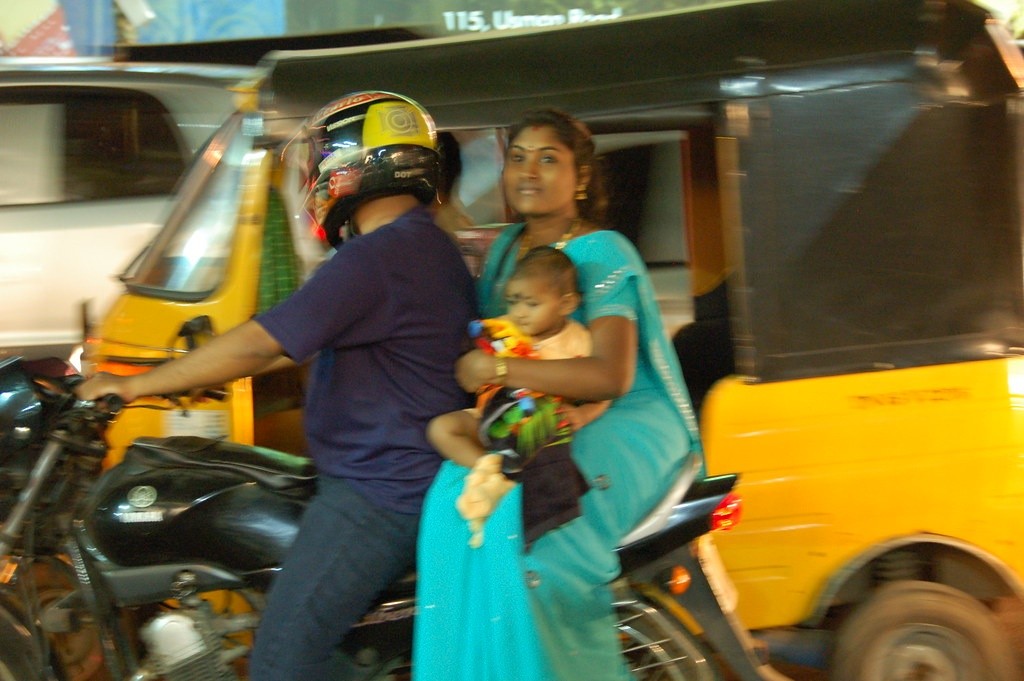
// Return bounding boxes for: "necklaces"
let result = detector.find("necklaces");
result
[515,213,582,259]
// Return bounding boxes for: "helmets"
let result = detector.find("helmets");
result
[277,91,439,245]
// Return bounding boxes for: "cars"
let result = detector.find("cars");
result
[4,57,510,448]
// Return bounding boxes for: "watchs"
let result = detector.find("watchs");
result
[495,355,510,388]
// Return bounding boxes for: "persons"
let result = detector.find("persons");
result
[74,90,481,681]
[411,103,694,681]
[424,245,618,523]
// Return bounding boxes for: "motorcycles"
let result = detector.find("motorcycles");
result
[0,315,789,680]
[39,2,1021,680]
